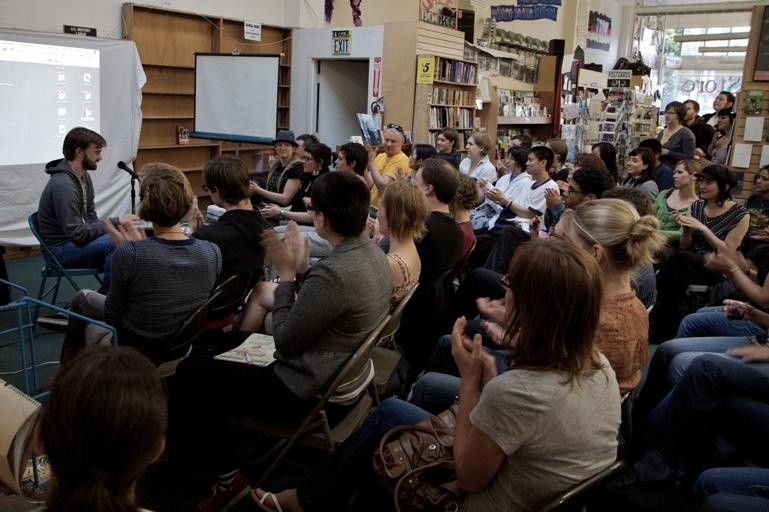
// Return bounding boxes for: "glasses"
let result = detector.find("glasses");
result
[498,273,515,293]
[386,122,404,136]
[567,185,591,196]
[547,224,566,242]
[302,154,321,162]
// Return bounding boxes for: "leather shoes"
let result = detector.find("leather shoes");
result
[608,473,682,494]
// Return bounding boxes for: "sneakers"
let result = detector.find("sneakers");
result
[197,470,253,512]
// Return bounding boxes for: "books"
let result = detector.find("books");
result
[428,56,478,160]
[214,332,277,368]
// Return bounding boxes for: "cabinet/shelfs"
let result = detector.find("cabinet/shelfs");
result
[599,100,658,179]
[476,76,552,172]
[575,67,607,154]
[121,2,292,212]
[411,52,479,161]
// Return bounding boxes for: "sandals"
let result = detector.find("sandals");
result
[249,486,283,512]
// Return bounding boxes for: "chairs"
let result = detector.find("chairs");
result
[646,304,654,315]
[621,391,632,407]
[251,314,392,487]
[542,460,623,512]
[465,235,476,259]
[370,282,419,405]
[435,265,455,303]
[28,211,104,331]
[152,275,237,369]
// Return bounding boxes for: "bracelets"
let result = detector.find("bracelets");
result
[506,201,513,208]
[280,210,285,216]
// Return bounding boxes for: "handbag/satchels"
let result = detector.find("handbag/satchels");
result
[370,399,462,512]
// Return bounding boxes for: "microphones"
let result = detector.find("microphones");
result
[117,161,140,180]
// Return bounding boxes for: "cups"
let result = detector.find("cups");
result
[728,302,744,320]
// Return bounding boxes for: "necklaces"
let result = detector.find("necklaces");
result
[154,231,185,236]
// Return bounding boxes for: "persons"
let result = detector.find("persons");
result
[35,90,768,512]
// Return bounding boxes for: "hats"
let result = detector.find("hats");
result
[271,128,300,149]
[693,164,738,187]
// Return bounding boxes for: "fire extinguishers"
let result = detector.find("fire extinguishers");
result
[371,96,384,131]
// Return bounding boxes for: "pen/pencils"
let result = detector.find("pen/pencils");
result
[245,351,252,365]
